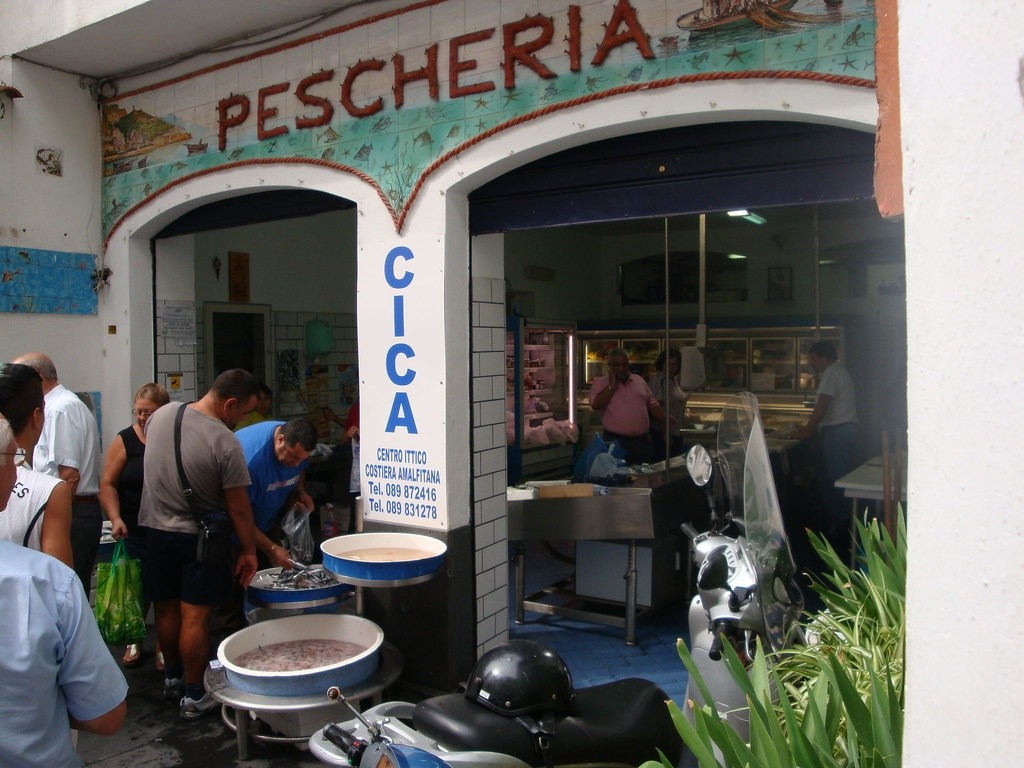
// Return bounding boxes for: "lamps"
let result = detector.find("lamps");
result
[525,264,556,282]
[726,209,752,218]
[743,212,766,225]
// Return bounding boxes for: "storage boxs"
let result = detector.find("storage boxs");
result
[254,699,361,751]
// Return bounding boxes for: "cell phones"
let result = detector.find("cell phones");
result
[616,373,622,379]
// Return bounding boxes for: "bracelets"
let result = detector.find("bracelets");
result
[608,385,614,391]
[268,544,277,557]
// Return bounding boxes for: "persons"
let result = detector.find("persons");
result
[12,352,102,606]
[0,411,128,768]
[0,361,75,572]
[589,347,677,465]
[646,350,688,461]
[99,369,361,719]
[790,344,861,544]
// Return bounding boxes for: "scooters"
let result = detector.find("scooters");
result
[305,390,806,768]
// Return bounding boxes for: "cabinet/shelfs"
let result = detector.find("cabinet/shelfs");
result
[507,317,860,480]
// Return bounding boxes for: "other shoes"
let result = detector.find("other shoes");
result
[163,678,185,702]
[180,691,219,721]
[156,652,164,671]
[123,644,142,666]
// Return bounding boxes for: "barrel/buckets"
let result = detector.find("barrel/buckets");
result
[319,531,448,580]
[216,613,385,698]
[244,563,354,626]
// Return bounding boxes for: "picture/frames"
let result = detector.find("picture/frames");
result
[768,266,793,302]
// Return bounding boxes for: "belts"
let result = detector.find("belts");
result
[76,496,99,505]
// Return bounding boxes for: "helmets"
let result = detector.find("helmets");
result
[459,639,577,718]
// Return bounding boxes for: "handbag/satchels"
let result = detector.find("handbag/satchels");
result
[197,514,235,569]
[96,536,147,644]
[279,504,314,563]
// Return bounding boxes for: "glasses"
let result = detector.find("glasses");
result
[0,448,27,466]
[132,409,151,419]
